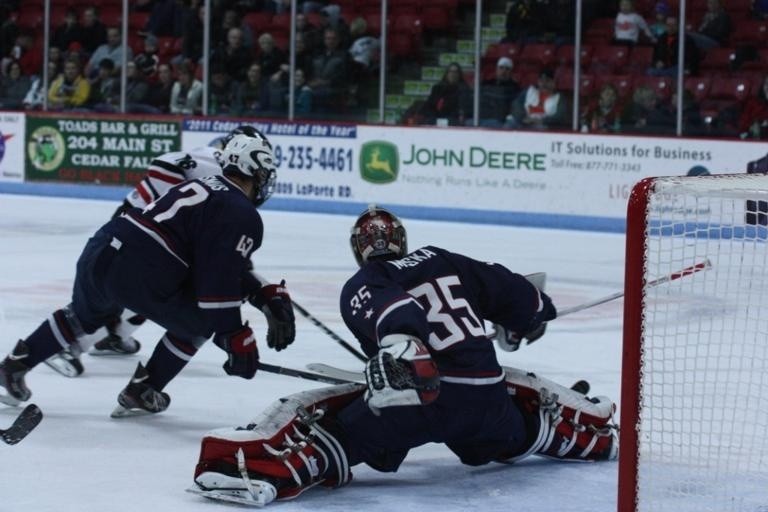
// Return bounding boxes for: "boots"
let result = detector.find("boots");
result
[0,339,37,401]
[118,361,170,412]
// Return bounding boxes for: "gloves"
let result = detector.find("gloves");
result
[255,279,295,351]
[219,320,259,379]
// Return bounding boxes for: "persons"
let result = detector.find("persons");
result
[1,1,768,140]
[193,203,620,508]
[0,135,296,414]
[59,125,272,376]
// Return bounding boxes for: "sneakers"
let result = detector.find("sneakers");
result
[94,333,139,353]
[52,348,82,375]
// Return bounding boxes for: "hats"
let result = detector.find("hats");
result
[497,57,513,68]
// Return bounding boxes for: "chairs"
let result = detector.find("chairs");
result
[1,1,767,139]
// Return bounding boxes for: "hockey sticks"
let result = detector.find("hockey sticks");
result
[305,260,714,382]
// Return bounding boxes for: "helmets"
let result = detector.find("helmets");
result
[351,207,407,267]
[219,126,276,207]
